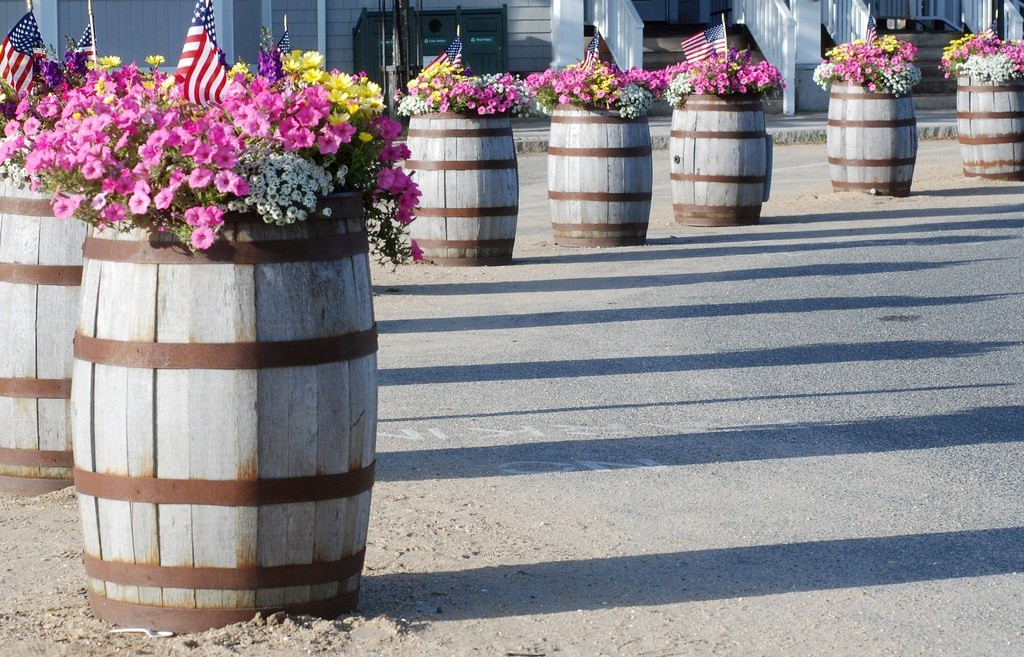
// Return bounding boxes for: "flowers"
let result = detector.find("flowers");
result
[812,34,922,98]
[393,42,788,120]
[938,27,1024,84]
[0,25,435,272]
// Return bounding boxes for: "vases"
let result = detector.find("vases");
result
[669,95,766,227]
[547,104,652,248]
[404,111,519,267]
[71,192,379,633]
[828,80,917,197]
[0,179,86,495]
[956,71,1024,180]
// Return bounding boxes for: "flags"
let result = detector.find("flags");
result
[681,24,725,64]
[867,14,879,50]
[177,0,232,108]
[425,36,462,71]
[0,11,49,97]
[274,27,290,58]
[580,31,601,71]
[77,15,98,64]
[985,18,998,39]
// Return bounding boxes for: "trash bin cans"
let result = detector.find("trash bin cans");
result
[350,3,509,102]
